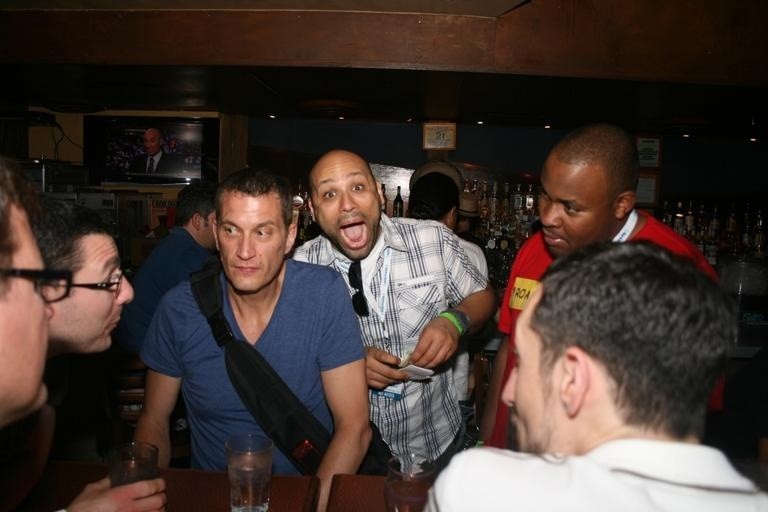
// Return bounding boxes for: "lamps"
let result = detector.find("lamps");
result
[544,118,551,129]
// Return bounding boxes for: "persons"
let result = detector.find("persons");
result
[733,343,768,463]
[115,179,218,355]
[1,164,170,511]
[410,171,460,229]
[420,240,768,511]
[455,191,486,254]
[129,166,375,512]
[287,147,498,481]
[22,187,135,356]
[474,117,739,452]
[128,127,182,173]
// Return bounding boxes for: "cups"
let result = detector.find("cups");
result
[113,443,157,486]
[384,456,434,511]
[226,431,274,510]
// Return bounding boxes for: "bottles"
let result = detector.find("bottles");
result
[393,185,404,216]
[665,199,768,271]
[462,176,540,284]
[282,179,314,241]
[379,184,387,213]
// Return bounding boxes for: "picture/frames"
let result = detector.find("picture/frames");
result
[422,120,458,151]
[633,171,661,209]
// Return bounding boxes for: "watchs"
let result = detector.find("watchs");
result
[439,308,472,337]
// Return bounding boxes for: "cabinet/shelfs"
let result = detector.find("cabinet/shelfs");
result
[67,184,188,279]
[24,106,249,184]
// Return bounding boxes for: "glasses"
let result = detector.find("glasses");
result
[349,263,369,316]
[3,269,122,302]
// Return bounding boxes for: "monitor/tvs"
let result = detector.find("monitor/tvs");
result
[81,109,223,195]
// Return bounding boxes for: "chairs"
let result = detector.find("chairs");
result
[458,351,496,451]
[96,350,192,458]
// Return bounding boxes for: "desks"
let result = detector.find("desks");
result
[326,473,436,512]
[14,461,320,512]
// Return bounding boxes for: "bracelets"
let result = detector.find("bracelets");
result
[476,440,484,448]
